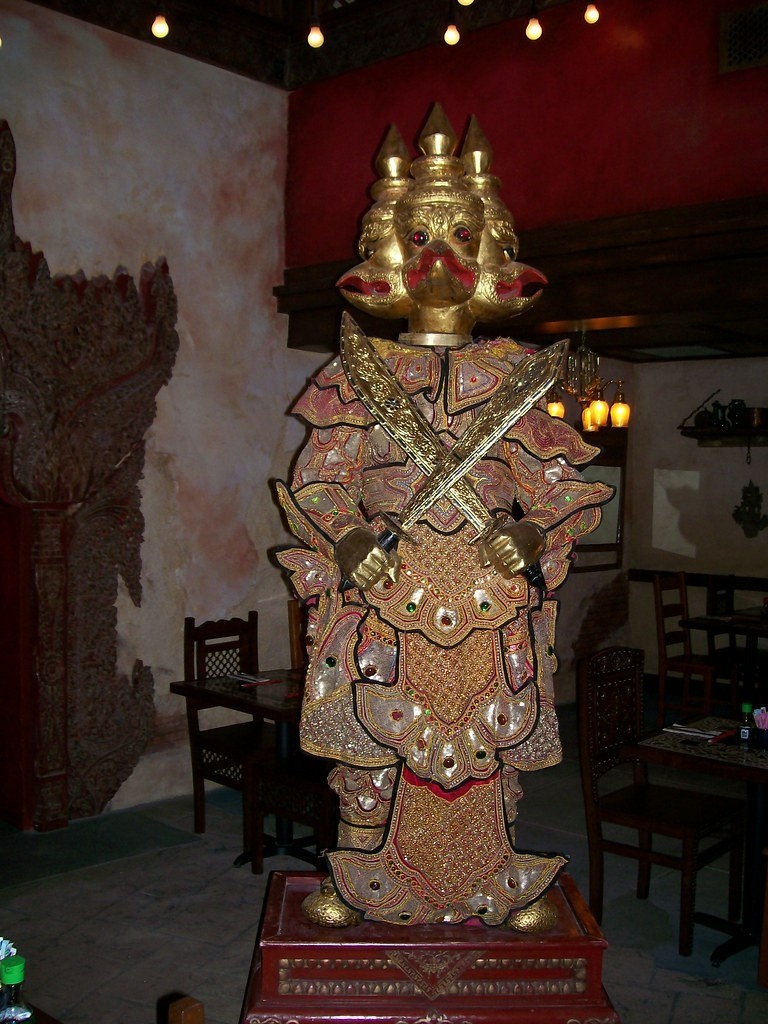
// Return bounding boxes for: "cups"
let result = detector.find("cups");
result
[754,726,768,752]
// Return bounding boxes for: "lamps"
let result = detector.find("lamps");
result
[538,330,631,433]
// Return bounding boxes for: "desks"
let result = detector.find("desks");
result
[679,606,768,720]
[171,669,334,874]
[620,714,768,967]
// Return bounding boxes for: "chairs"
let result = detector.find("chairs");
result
[184,611,258,848]
[579,645,743,957]
[710,574,767,715]
[653,572,738,728]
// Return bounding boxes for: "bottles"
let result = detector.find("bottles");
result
[0,956,36,1024]
[736,703,755,753]
[710,401,720,427]
[719,405,730,427]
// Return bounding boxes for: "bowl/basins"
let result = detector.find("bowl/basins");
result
[745,407,768,428]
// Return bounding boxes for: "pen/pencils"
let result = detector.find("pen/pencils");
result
[707,729,737,742]
